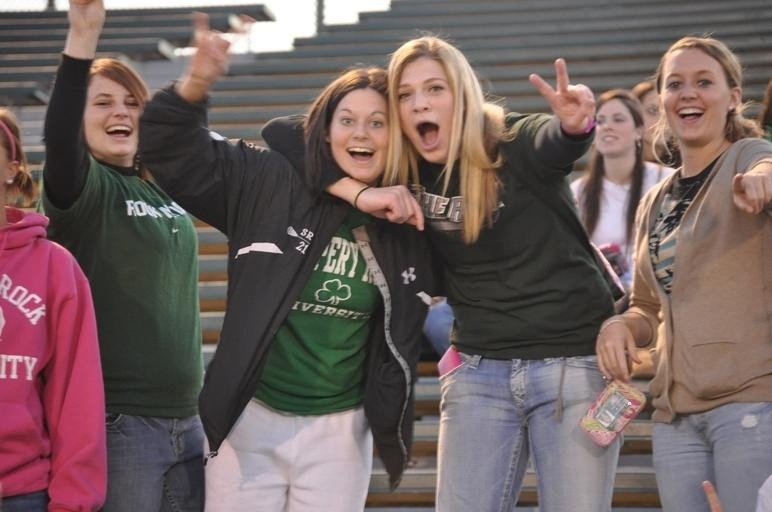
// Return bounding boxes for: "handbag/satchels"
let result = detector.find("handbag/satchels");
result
[579,378,647,449]
[588,241,629,314]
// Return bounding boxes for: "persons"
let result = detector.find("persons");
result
[423,297,454,360]
[262,36,630,512]
[564,38,771,512]
[0,115,108,512]
[42,1,210,511]
[134,8,434,512]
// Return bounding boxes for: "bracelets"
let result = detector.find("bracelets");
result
[355,185,372,209]
